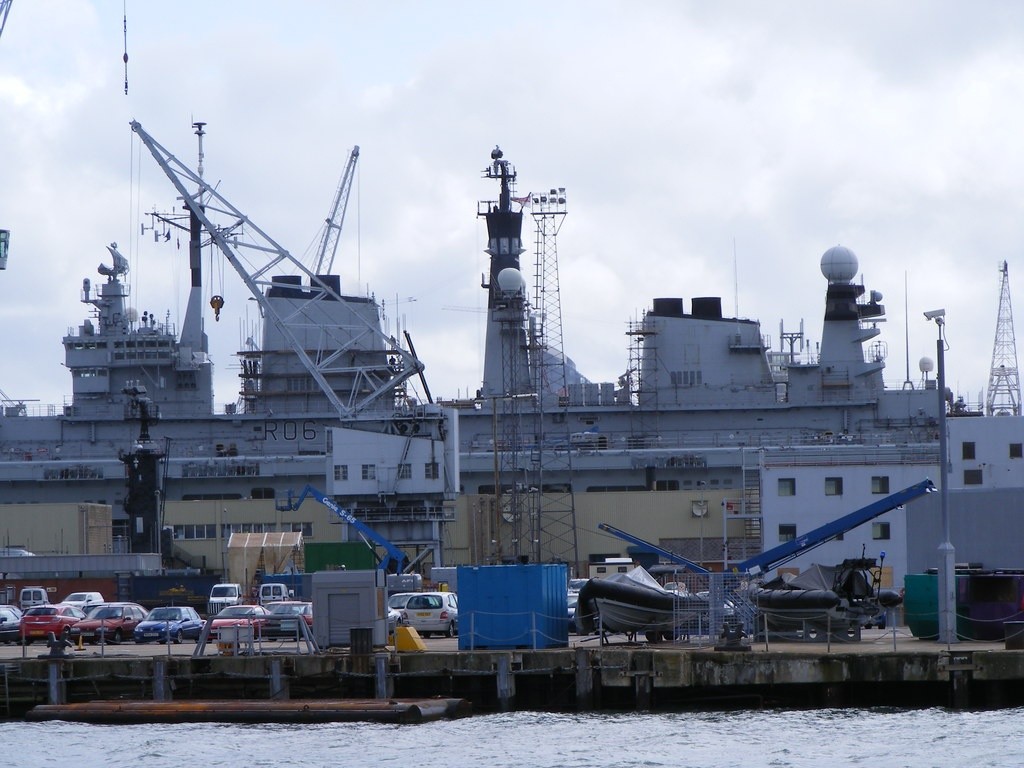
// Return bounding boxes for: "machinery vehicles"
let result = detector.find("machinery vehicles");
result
[262,484,421,595]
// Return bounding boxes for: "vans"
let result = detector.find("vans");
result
[208,583,244,613]
[258,583,294,608]
[19,585,49,612]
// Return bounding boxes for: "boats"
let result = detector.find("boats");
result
[574,565,708,631]
[745,542,903,630]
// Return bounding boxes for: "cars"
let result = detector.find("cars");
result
[565,577,589,630]
[388,593,424,617]
[263,602,281,612]
[401,592,458,638]
[387,606,403,632]
[0,605,24,644]
[81,603,105,613]
[661,580,740,614]
[265,601,313,641]
[202,605,271,643]
[19,604,88,645]
[133,606,205,645]
[70,603,149,645]
[61,591,105,609]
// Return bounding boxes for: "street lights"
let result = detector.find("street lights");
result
[925,306,959,642]
